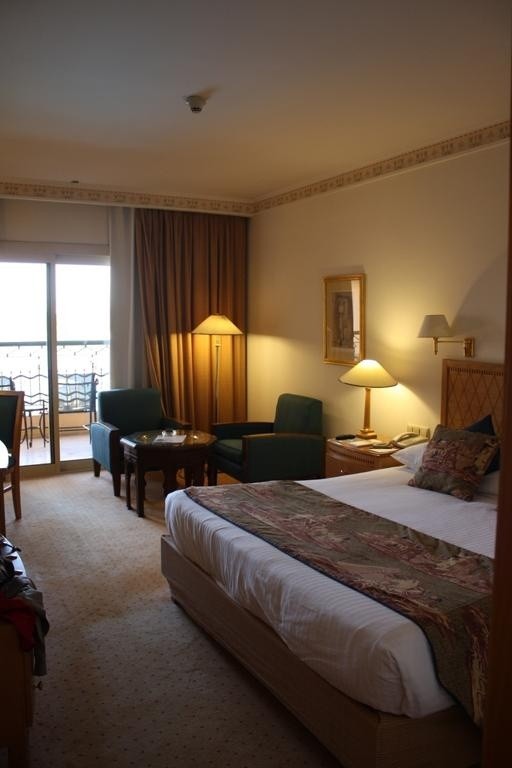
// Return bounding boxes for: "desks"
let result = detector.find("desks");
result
[19,407,50,445]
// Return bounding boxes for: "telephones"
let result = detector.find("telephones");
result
[372,432,430,448]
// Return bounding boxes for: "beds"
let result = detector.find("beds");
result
[161,360,508,768]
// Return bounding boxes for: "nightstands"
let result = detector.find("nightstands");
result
[324,435,402,477]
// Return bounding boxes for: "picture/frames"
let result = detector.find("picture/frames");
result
[322,273,366,366]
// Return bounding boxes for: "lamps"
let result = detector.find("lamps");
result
[337,359,398,439]
[418,315,474,359]
[190,314,244,422]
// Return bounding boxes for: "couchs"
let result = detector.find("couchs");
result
[210,392,326,483]
[90,387,192,496]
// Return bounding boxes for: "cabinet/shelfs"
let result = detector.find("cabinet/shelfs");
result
[1,536,43,768]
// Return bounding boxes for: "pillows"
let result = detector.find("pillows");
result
[460,413,500,473]
[391,439,428,472]
[407,423,503,501]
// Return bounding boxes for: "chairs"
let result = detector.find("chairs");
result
[1,376,14,391]
[0,391,25,536]
[56,380,97,434]
